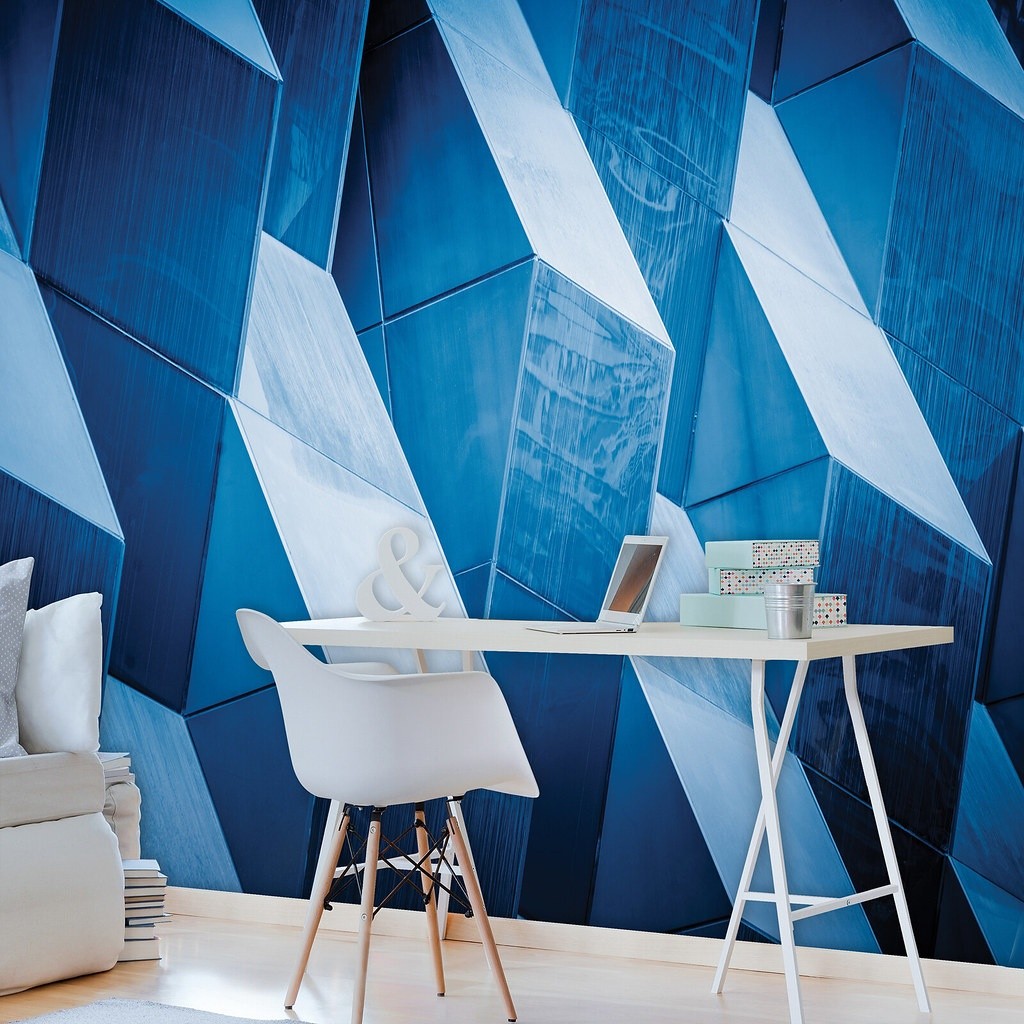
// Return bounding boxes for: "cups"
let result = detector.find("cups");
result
[764,582,819,637]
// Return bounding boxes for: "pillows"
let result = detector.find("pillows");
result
[0,556,36,760]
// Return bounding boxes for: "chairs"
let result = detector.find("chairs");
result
[237,606,540,1024]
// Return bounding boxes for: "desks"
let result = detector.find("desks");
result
[282,617,956,1024]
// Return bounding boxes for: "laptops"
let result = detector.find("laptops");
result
[526,536,669,635]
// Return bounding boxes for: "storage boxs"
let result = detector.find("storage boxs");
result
[679,593,848,630]
[708,568,818,595]
[707,539,823,568]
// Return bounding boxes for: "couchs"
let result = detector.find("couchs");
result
[0,588,126,997]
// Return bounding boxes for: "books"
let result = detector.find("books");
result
[98,752,135,790]
[118,860,173,962]
